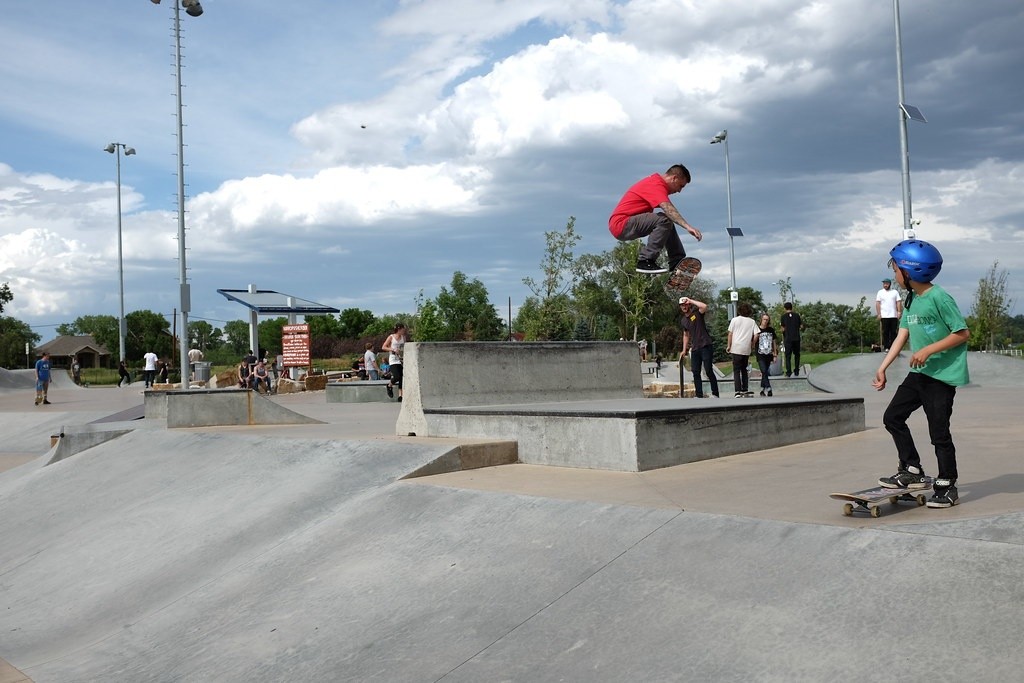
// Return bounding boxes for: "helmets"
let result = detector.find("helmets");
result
[890,239,943,284]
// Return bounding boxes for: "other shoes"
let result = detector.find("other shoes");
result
[760,391,766,396]
[36,402,38,406]
[734,391,748,398]
[386,384,393,398]
[398,396,402,402]
[767,390,772,397]
[710,394,719,399]
[884,349,889,354]
[43,400,52,404]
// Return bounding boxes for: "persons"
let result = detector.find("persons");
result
[144,348,168,388]
[870,239,970,508]
[237,344,282,394]
[35,352,51,405]
[71,358,80,385]
[352,324,406,401]
[638,339,661,370]
[678,297,719,398]
[188,345,203,381]
[726,302,803,397]
[608,164,702,273]
[117,359,130,388]
[875,278,902,354]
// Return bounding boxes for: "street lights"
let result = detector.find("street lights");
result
[709,129,744,318]
[103,141,136,385]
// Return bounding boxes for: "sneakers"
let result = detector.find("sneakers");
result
[878,462,927,488]
[635,260,668,274]
[926,478,960,508]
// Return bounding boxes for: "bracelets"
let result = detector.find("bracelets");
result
[774,356,777,357]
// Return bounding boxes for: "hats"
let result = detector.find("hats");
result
[679,297,687,304]
[881,279,891,283]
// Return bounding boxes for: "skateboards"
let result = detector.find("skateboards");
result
[676,354,684,398]
[740,391,755,398]
[828,475,935,518]
[35,379,44,404]
[662,256,703,302]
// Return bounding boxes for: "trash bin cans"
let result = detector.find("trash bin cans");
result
[50,433,59,448]
[194,362,212,382]
[769,348,783,376]
